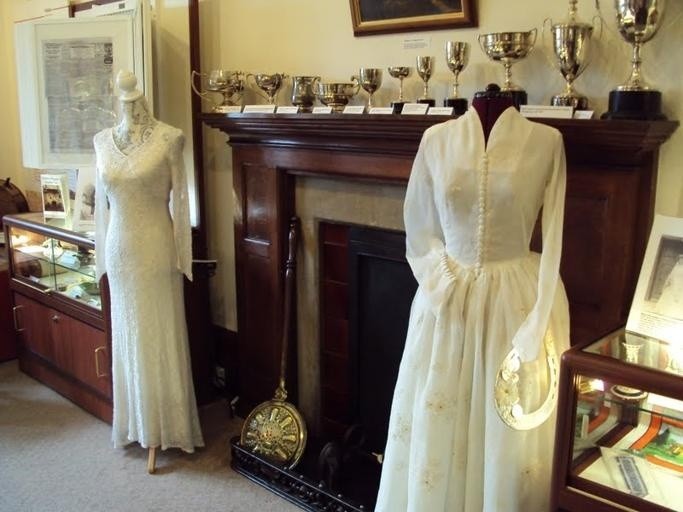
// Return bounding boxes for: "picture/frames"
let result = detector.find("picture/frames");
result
[624,212,683,348]
[349,0,478,39]
[32,14,135,166]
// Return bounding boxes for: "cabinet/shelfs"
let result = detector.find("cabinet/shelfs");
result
[554,322,683,507]
[197,110,683,512]
[3,208,117,426]
